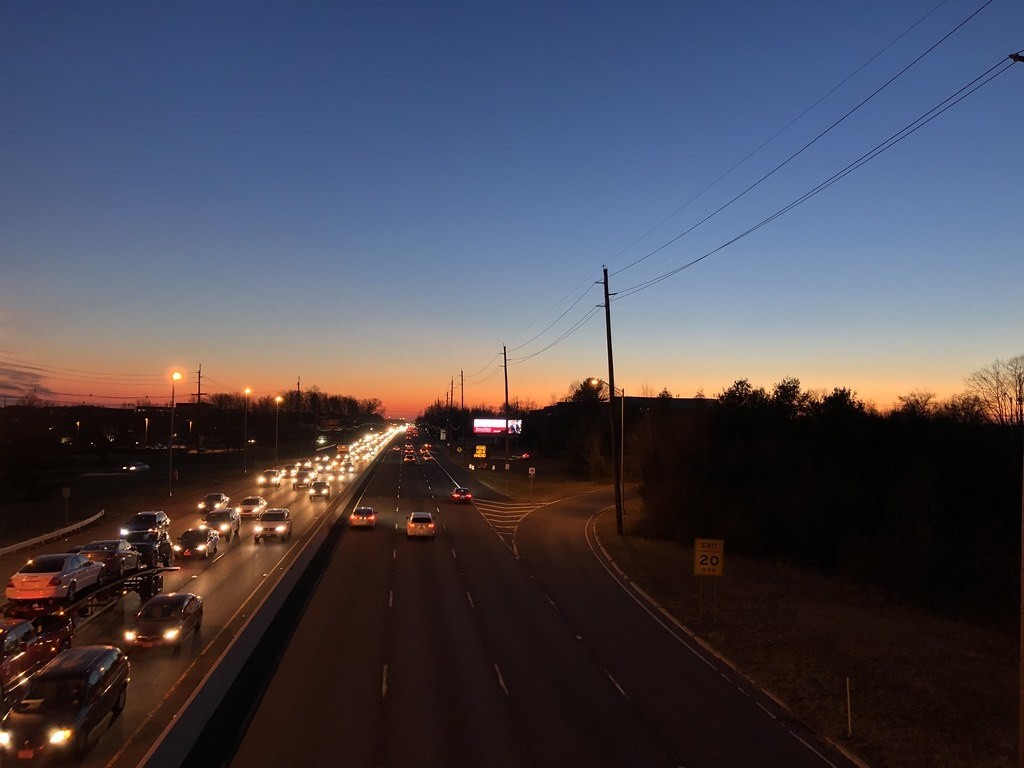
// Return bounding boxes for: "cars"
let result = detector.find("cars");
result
[174,528,220,558]
[77,539,143,578]
[120,511,170,540]
[348,507,378,529]
[254,508,293,541]
[393,430,433,463]
[197,492,241,541]
[0,644,131,768]
[236,444,376,516]
[121,530,173,568]
[406,512,436,539]
[123,462,150,473]
[450,488,472,503]
[125,593,203,651]
[5,553,106,603]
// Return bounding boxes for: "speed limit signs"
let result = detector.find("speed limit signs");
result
[692,538,724,576]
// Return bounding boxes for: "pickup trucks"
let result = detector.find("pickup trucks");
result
[0,614,76,707]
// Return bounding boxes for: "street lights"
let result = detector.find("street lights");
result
[592,379,625,514]
[169,372,182,496]
[244,388,281,473]
